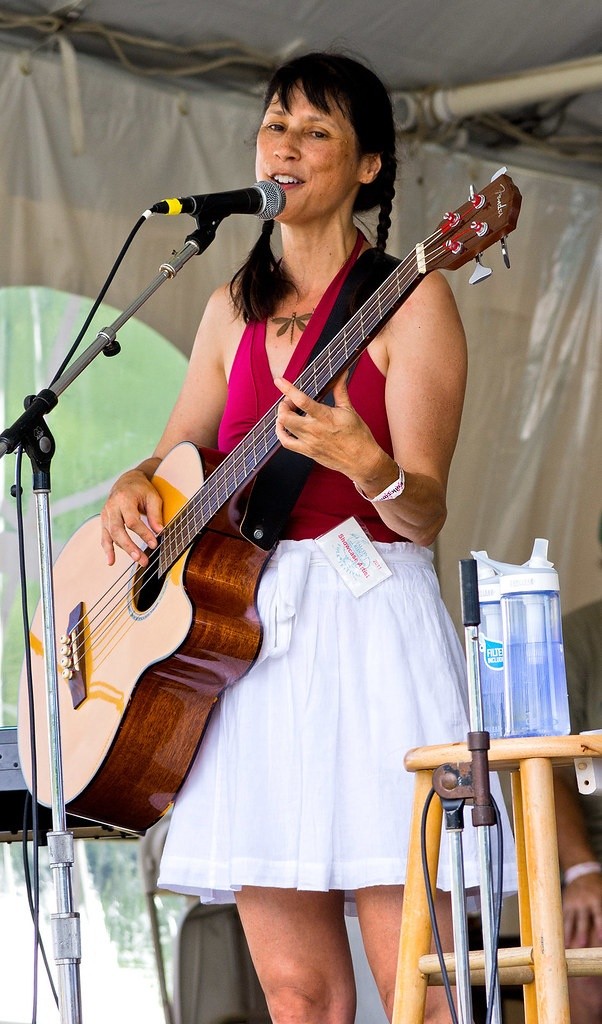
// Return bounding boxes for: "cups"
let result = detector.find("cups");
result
[473,548,508,739]
[469,538,572,738]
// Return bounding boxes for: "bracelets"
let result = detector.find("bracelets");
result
[354,461,404,502]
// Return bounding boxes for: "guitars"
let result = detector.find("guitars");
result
[14,162,525,839]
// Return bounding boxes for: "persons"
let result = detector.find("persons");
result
[100,52,519,1024]
[552,510,601,1024]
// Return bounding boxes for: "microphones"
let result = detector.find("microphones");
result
[154,180,286,221]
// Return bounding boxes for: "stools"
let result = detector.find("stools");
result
[388,726,602,1024]
